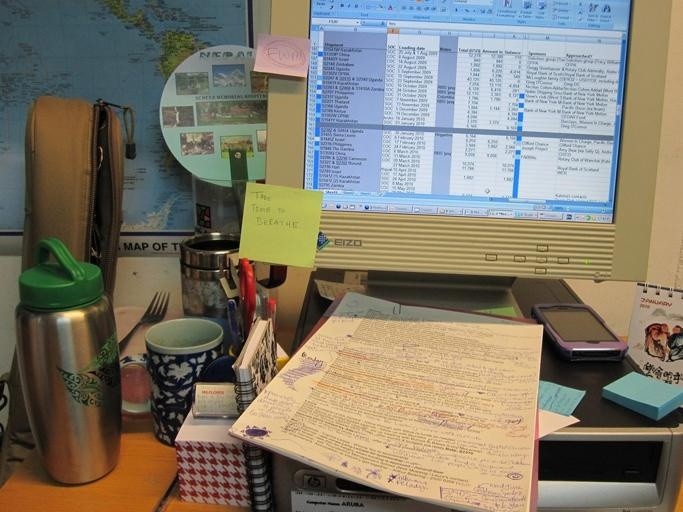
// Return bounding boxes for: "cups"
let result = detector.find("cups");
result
[179,231,256,357]
[143,316,226,447]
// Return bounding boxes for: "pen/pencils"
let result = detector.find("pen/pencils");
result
[226,297,278,357]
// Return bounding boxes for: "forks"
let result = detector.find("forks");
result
[118,290,171,359]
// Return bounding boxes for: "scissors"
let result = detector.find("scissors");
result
[239,259,256,339]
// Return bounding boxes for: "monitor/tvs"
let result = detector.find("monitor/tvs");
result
[264,1,673,353]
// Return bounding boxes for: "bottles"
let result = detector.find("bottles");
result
[14,235,123,487]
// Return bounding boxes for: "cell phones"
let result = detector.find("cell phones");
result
[532,302,629,365]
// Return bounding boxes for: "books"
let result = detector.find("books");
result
[227,290,544,512]
[232,316,281,511]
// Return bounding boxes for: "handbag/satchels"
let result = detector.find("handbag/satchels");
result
[9,95,134,429]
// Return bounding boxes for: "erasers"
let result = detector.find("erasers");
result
[601,372,683,421]
